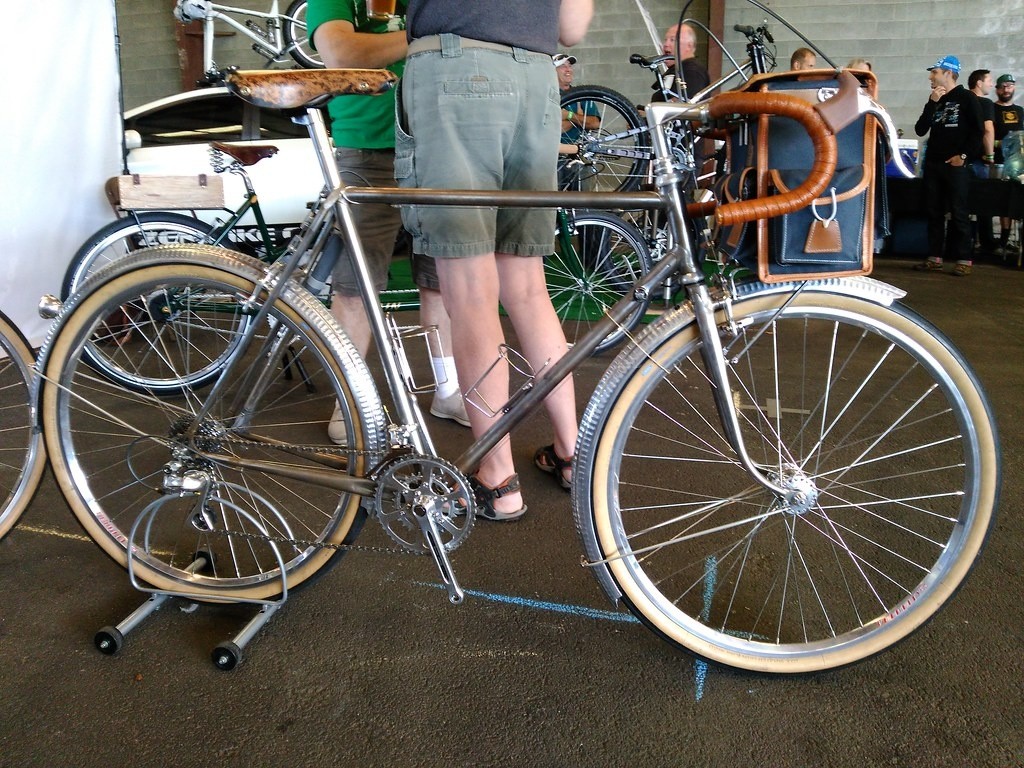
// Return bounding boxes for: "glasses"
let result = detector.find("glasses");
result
[994,83,1015,88]
[554,55,569,61]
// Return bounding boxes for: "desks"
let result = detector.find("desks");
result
[970,177,1024,252]
[884,175,943,261]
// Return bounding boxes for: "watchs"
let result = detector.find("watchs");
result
[959,153,968,163]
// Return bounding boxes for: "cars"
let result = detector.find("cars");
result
[122,86,409,260]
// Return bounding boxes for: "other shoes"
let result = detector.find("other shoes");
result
[912,260,943,271]
[329,405,346,444]
[430,386,470,426]
[953,264,970,276]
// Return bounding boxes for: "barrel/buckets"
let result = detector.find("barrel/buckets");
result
[885,139,919,177]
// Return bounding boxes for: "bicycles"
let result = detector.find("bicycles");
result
[54,142,652,399]
[173,0,331,77]
[549,20,780,304]
[1,307,51,544]
[28,66,1001,673]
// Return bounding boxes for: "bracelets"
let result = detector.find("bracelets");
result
[567,111,573,121]
[986,154,994,159]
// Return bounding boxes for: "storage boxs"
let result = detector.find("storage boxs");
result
[884,137,920,178]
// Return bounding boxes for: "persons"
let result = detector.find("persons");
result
[913,55,985,276]
[306,0,471,443]
[551,54,603,210]
[850,60,871,72]
[371,0,594,522]
[968,70,995,257]
[994,74,1024,256]
[652,24,710,245]
[792,48,816,72]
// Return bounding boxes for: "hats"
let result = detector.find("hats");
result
[995,74,1015,86]
[926,56,960,72]
[551,53,578,67]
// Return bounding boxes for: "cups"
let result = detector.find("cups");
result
[994,164,1004,179]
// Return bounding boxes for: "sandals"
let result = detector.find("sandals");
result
[443,467,528,519]
[535,443,575,488]
